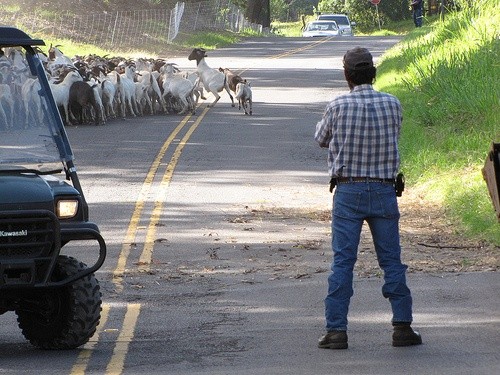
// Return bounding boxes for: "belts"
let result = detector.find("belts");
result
[338,176,394,182]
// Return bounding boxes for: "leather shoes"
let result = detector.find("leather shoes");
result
[318,330,349,349]
[392,325,422,346]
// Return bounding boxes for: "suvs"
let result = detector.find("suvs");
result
[318,12,356,37]
[0,26,101,352]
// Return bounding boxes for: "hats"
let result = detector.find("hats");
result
[343,46,373,70]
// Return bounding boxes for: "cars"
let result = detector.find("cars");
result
[301,20,345,38]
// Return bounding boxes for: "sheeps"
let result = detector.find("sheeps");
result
[0,43,253,130]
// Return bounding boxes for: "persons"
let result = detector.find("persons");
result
[410,0,422,27]
[313,47,423,349]
[326,24,334,30]
